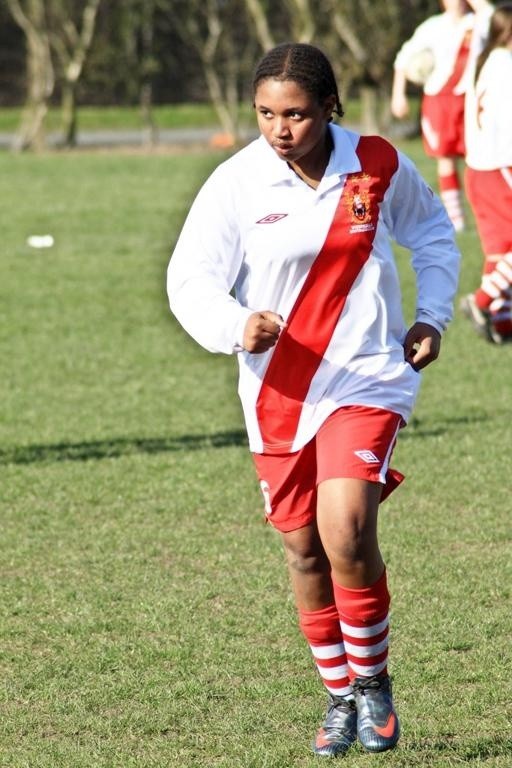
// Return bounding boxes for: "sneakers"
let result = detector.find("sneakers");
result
[354,676,402,753]
[310,691,359,756]
[460,293,499,344]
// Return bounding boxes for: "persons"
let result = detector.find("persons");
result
[457,2,512,346]
[162,42,463,764]
[387,0,498,233]
